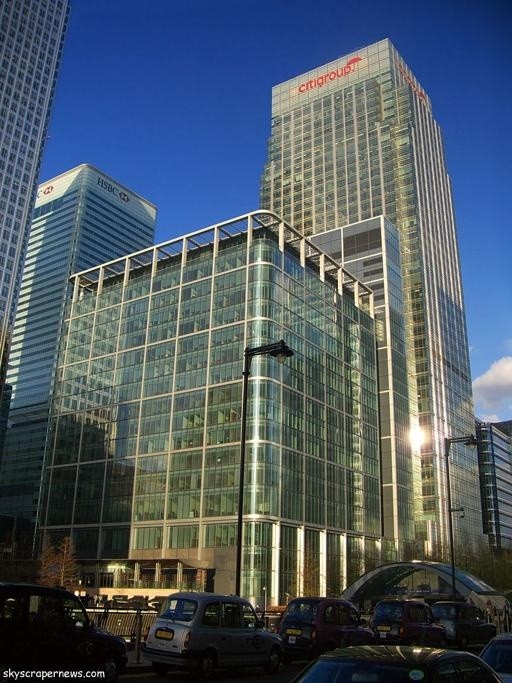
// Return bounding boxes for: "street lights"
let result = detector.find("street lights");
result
[78,580,82,600]
[236,338,295,597]
[444,434,481,600]
[262,585,268,615]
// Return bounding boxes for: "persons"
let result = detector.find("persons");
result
[469,598,474,604]
[502,599,512,631]
[483,599,492,620]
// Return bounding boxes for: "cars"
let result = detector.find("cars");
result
[274,596,375,665]
[477,633,512,682]
[5,592,260,613]
[428,600,497,650]
[141,592,286,682]
[284,644,503,683]
[369,599,447,648]
[1,582,129,682]
